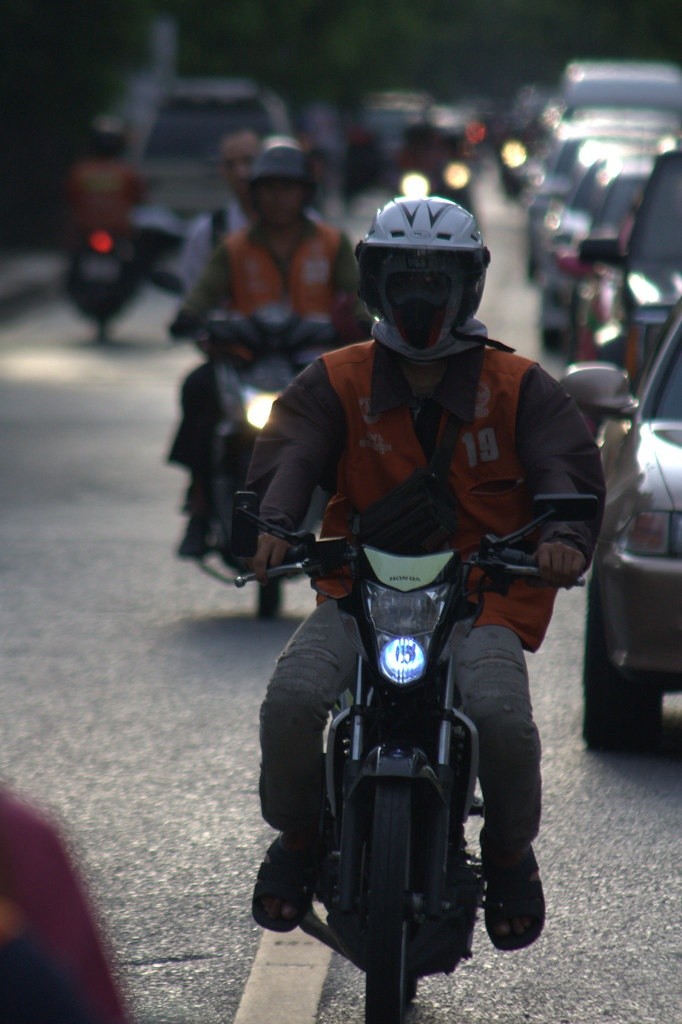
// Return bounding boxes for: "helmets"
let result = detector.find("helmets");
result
[354,195,490,328]
[248,132,319,190]
[78,114,125,136]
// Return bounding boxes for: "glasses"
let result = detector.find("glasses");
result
[385,271,451,306]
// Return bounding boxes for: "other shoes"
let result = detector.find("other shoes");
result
[178,518,209,556]
[251,837,315,933]
[481,844,545,951]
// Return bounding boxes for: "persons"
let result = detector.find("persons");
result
[169,140,377,555]
[56,119,148,295]
[0,786,131,1024]
[244,195,605,950]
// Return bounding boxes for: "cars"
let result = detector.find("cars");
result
[56,47,681,748]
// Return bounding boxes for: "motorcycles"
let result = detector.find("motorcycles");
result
[146,269,344,621]
[224,488,598,1024]
